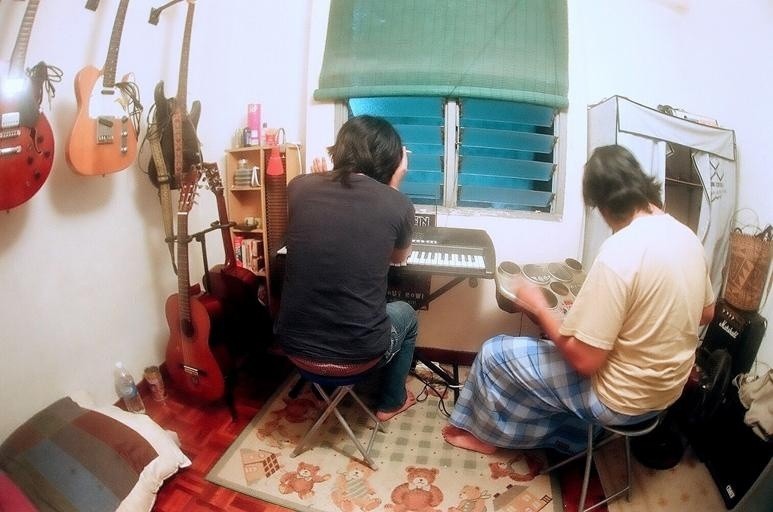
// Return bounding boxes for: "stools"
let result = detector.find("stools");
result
[288,355,389,472]
[539,414,660,512]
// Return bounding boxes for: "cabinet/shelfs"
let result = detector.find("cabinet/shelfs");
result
[581,96,736,349]
[225,144,303,314]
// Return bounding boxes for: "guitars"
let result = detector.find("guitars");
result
[150,1,204,188]
[0,1,56,212]
[202,163,256,349]
[66,0,136,177]
[164,182,230,401]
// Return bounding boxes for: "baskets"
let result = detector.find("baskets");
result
[724,225,773,312]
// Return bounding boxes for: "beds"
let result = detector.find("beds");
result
[1,391,192,511]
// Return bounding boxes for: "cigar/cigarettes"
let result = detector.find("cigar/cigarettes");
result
[405,149,412,153]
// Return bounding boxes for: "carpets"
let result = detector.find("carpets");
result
[204,360,564,512]
[592,440,727,512]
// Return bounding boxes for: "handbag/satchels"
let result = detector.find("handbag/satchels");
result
[731,369,773,442]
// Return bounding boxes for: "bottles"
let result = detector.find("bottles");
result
[112,360,147,415]
[260,121,268,146]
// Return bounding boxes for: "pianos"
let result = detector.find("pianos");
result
[277,225,496,279]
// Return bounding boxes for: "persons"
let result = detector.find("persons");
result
[273,115,417,421]
[443,145,716,455]
